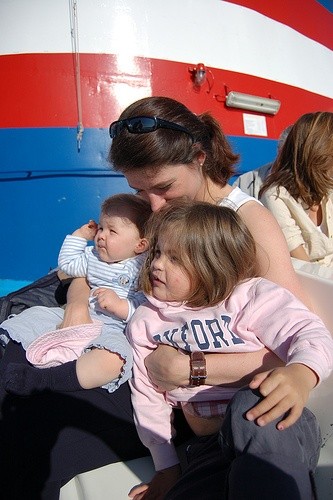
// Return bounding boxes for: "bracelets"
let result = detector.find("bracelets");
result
[188,349,206,389]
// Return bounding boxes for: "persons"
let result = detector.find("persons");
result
[257,111,332,286]
[233,124,298,201]
[0,96,321,500]
[123,201,332,500]
[3,191,152,395]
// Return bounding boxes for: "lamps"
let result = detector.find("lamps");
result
[189,63,215,93]
[218,83,280,115]
